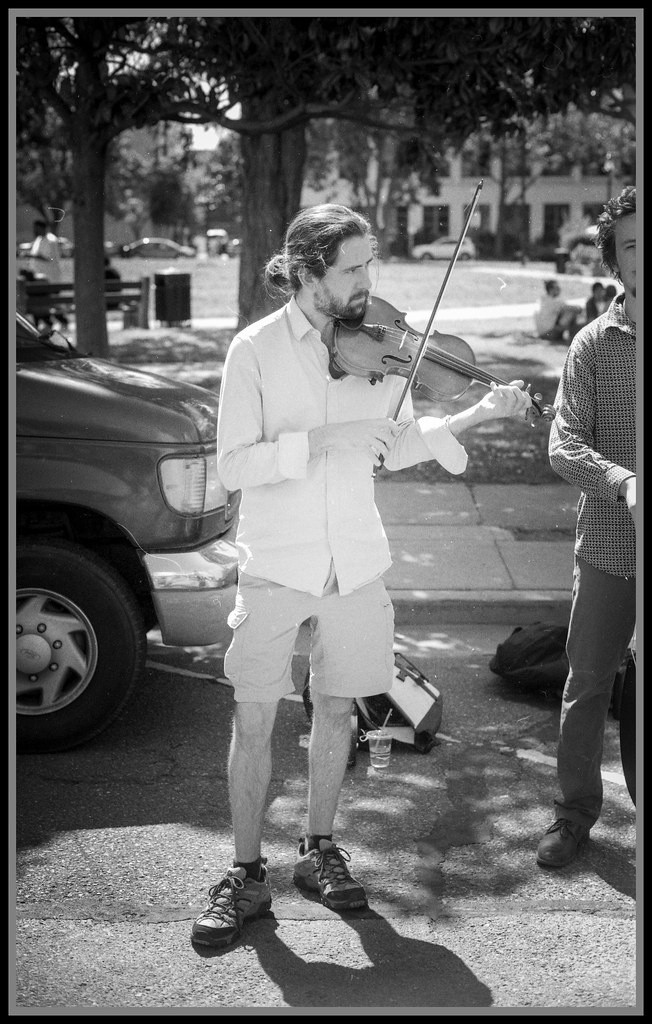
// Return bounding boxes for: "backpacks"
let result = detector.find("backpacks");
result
[303,649,444,751]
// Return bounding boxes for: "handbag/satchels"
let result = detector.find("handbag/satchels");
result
[490,619,634,716]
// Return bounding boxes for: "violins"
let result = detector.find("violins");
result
[335,293,560,431]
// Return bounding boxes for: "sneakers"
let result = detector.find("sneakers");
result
[190,858,272,947]
[293,837,368,909]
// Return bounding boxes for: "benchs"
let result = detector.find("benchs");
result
[16,276,150,329]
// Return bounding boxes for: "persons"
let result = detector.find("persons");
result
[535,280,616,344]
[103,258,122,310]
[20,222,69,333]
[188,207,532,948]
[537,187,635,866]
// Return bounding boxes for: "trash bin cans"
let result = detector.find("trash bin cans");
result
[554,249,571,273]
[153,270,192,323]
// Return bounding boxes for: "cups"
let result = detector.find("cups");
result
[366,730,393,768]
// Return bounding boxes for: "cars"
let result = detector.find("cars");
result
[119,235,197,261]
[411,235,477,262]
[16,307,247,760]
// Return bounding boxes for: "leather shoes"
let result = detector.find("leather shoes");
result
[536,818,587,869]
